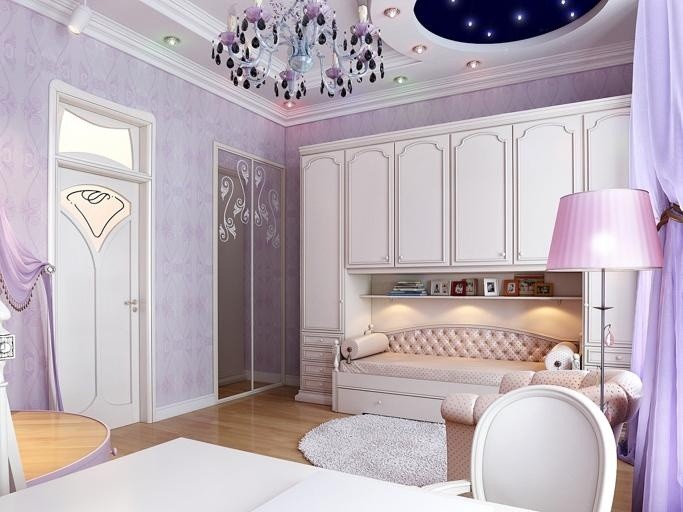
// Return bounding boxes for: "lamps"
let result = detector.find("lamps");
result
[67,0,91,35]
[208,1,386,103]
[544,188,664,415]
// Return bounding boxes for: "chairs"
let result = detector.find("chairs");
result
[421,384,619,511]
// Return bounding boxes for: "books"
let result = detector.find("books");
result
[387,281,429,296]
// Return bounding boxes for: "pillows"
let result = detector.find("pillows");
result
[543,340,578,372]
[339,333,389,364]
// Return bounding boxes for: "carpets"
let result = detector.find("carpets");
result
[296,414,447,488]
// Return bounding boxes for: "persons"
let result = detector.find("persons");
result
[488,283,494,292]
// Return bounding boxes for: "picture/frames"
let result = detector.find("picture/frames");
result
[427,274,555,297]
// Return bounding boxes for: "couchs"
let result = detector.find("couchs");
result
[331,323,582,420]
[440,368,644,500]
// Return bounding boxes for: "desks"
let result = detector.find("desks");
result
[0,432,540,512]
[6,407,112,489]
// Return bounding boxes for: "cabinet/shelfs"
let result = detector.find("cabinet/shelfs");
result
[297,148,370,396]
[583,106,638,371]
[450,114,583,268]
[346,133,450,267]
